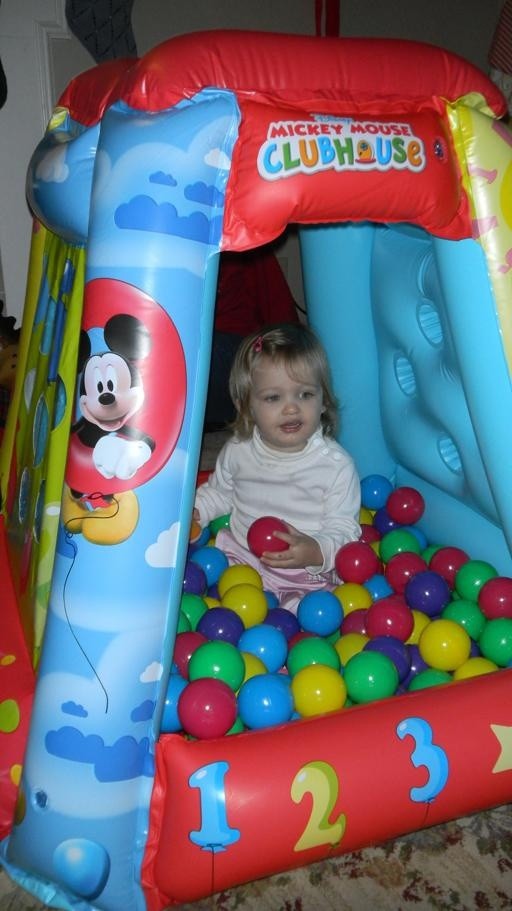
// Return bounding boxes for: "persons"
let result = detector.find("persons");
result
[189,323,363,615]
